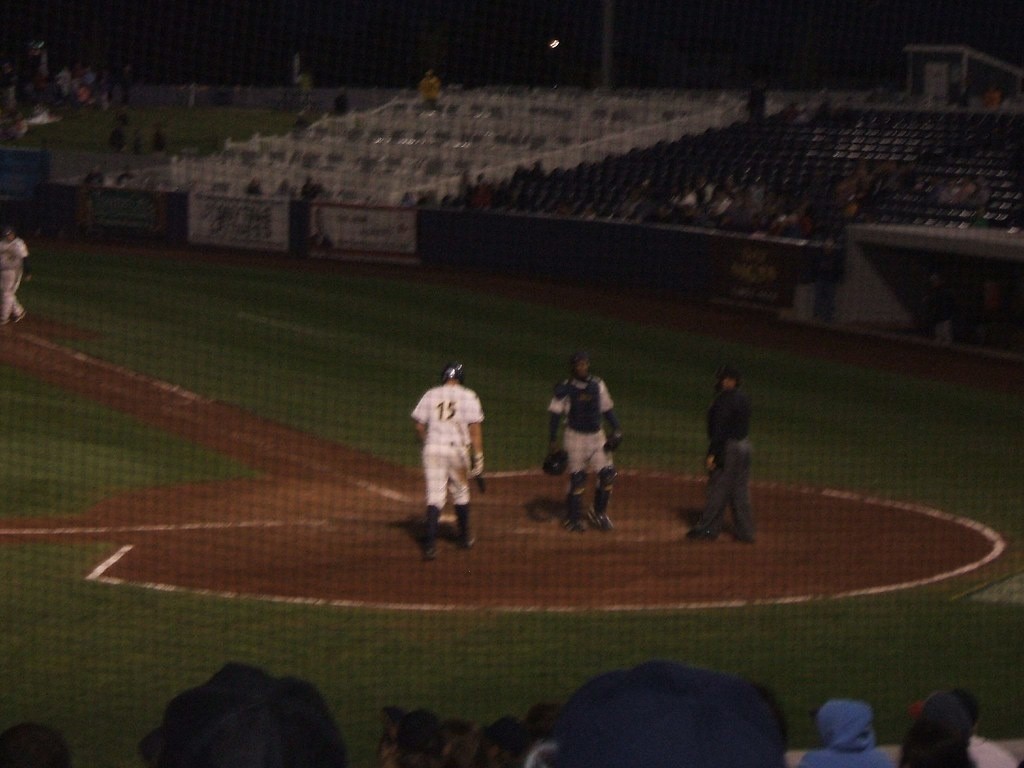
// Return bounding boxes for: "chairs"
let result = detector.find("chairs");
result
[67,84,1024,240]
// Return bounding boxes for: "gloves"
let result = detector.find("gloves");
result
[467,453,484,476]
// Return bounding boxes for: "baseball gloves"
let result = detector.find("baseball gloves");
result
[542,452,568,475]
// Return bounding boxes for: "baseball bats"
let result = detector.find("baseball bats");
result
[469,452,487,493]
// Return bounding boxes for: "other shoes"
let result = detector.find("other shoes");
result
[686,528,715,539]
[424,549,438,561]
[14,309,27,322]
[461,534,475,548]
[589,506,613,531]
[0,319,10,325]
[567,513,585,532]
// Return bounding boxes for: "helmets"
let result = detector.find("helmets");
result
[441,364,463,382]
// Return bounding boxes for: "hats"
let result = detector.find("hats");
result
[572,353,589,365]
[558,660,784,768]
[141,663,345,768]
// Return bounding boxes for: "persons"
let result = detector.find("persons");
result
[108,119,166,156]
[984,82,1003,111]
[547,353,622,531]
[898,688,1019,768]
[798,696,891,768]
[0,46,133,110]
[557,659,788,768]
[245,176,323,201]
[334,87,349,116]
[84,164,129,187]
[400,158,988,238]
[0,222,29,323]
[410,362,486,560]
[795,236,845,323]
[136,656,345,768]
[421,68,439,112]
[748,78,765,117]
[926,275,952,347]
[685,368,756,543]
[373,705,562,768]
